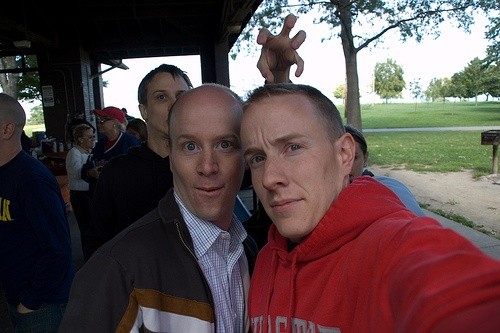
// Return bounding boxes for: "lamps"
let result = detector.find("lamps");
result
[12,24,32,49]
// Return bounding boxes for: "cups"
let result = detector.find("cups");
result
[65,143,71,150]
[58,142,63,153]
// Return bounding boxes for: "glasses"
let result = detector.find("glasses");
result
[100,118,113,125]
[79,135,96,139]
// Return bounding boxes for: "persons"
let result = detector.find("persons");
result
[59,83,260,333]
[344,125,426,218]
[0,92,78,333]
[240,82,499,333]
[99,14,306,247]
[81,107,137,249]
[66,125,95,261]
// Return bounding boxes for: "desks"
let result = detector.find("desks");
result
[37,152,68,168]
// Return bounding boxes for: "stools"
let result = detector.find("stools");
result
[481,129,500,174]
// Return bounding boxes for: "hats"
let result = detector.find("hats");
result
[93,107,125,123]
